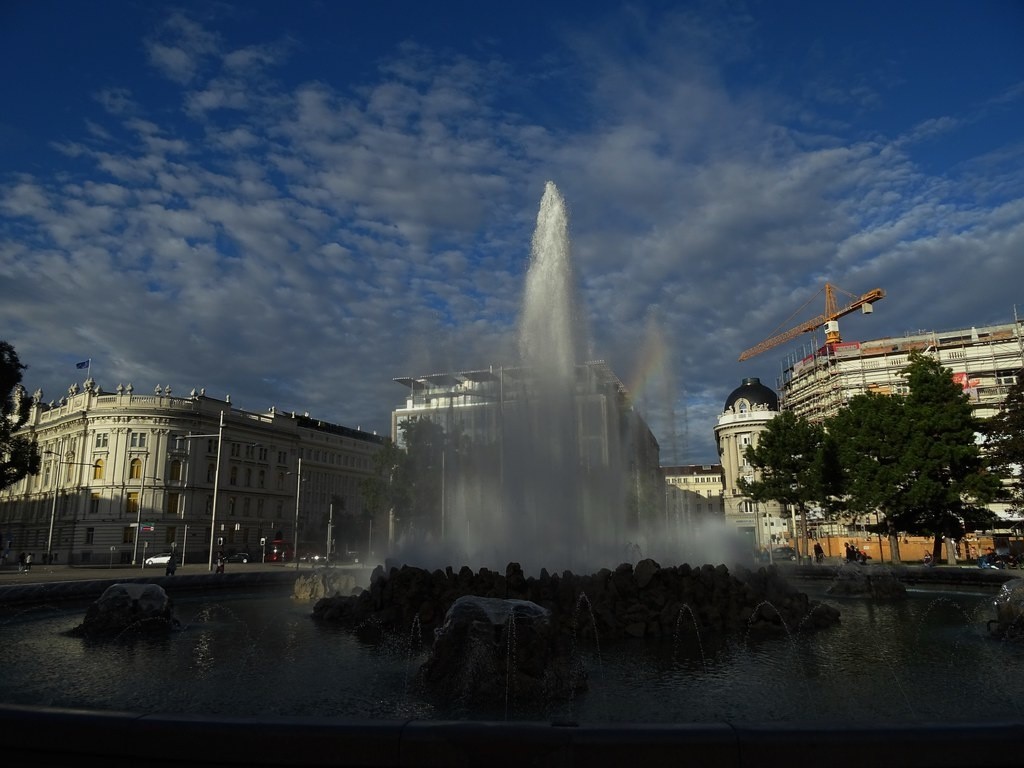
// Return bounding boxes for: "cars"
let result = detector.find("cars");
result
[225,553,250,564]
[146,552,172,565]
[763,547,797,561]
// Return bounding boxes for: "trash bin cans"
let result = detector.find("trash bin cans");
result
[802,554,811,566]
[42,553,53,565]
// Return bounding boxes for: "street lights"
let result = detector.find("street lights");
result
[175,410,226,572]
[43,438,102,567]
[286,457,309,564]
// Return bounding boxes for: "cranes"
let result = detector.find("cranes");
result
[737,282,885,363]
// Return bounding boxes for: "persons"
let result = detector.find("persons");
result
[987,547,997,563]
[165,553,177,576]
[845,543,866,565]
[17,552,33,575]
[214,551,224,573]
[924,550,933,568]
[814,543,823,563]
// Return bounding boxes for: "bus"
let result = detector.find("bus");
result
[265,540,307,562]
